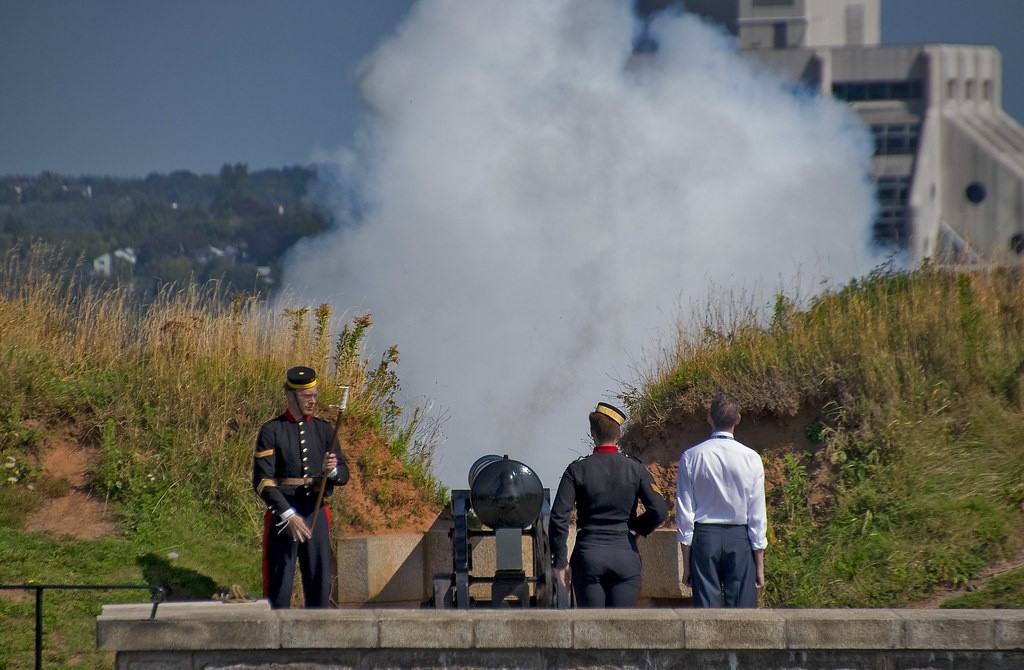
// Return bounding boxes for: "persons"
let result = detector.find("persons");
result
[548,402,669,610]
[674,392,768,608]
[253,366,349,609]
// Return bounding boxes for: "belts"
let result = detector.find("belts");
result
[280,477,318,486]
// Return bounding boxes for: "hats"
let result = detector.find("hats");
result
[282,366,318,391]
[596,402,627,427]
